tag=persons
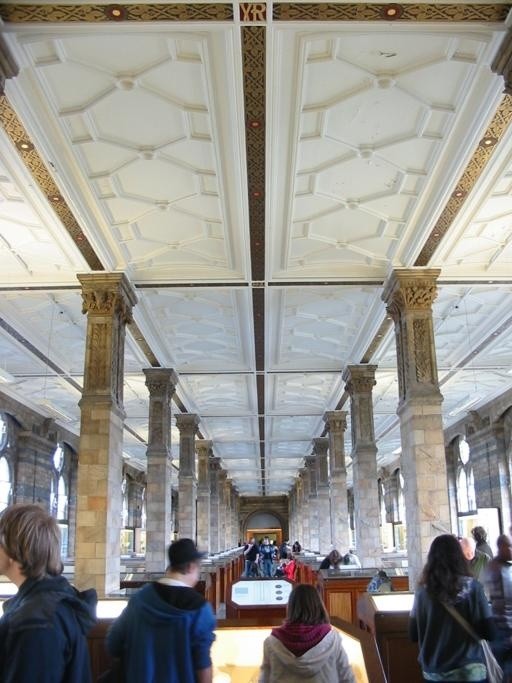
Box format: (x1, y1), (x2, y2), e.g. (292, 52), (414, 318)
(470, 525), (494, 560)
(237, 535), (360, 578)
(408, 534), (498, 682)
(102, 536), (217, 682)
(1, 505), (102, 682)
(367, 569), (395, 592)
(460, 537), (491, 581)
(477, 534), (512, 682)
(257, 583), (359, 682)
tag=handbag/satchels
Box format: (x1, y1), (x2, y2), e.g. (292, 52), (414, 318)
(479, 639), (505, 683)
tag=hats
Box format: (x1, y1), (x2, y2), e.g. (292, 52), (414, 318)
(169, 539), (207, 566)
